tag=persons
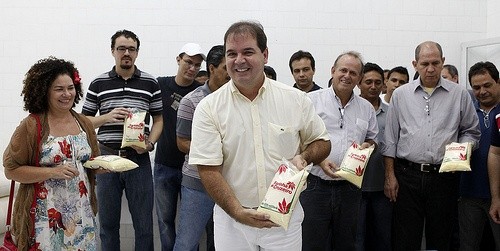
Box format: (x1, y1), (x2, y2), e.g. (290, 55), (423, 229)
(194, 71), (216, 251)
(172, 45), (231, 251)
(264, 66), (276, 81)
(153, 42), (208, 251)
(188, 21), (332, 251)
(289, 41), (500, 251)
(81, 29), (164, 251)
(3, 58), (112, 251)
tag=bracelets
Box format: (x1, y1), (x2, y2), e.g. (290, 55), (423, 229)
(148, 140), (155, 151)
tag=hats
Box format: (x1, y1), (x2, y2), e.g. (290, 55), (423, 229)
(178, 43), (207, 61)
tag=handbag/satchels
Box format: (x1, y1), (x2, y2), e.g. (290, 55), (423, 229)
(0, 230), (40, 251)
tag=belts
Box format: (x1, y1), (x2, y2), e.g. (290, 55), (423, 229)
(99, 143), (137, 157)
(402, 159), (441, 175)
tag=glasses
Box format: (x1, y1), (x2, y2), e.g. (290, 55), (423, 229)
(112, 46), (139, 53)
(179, 55), (202, 69)
